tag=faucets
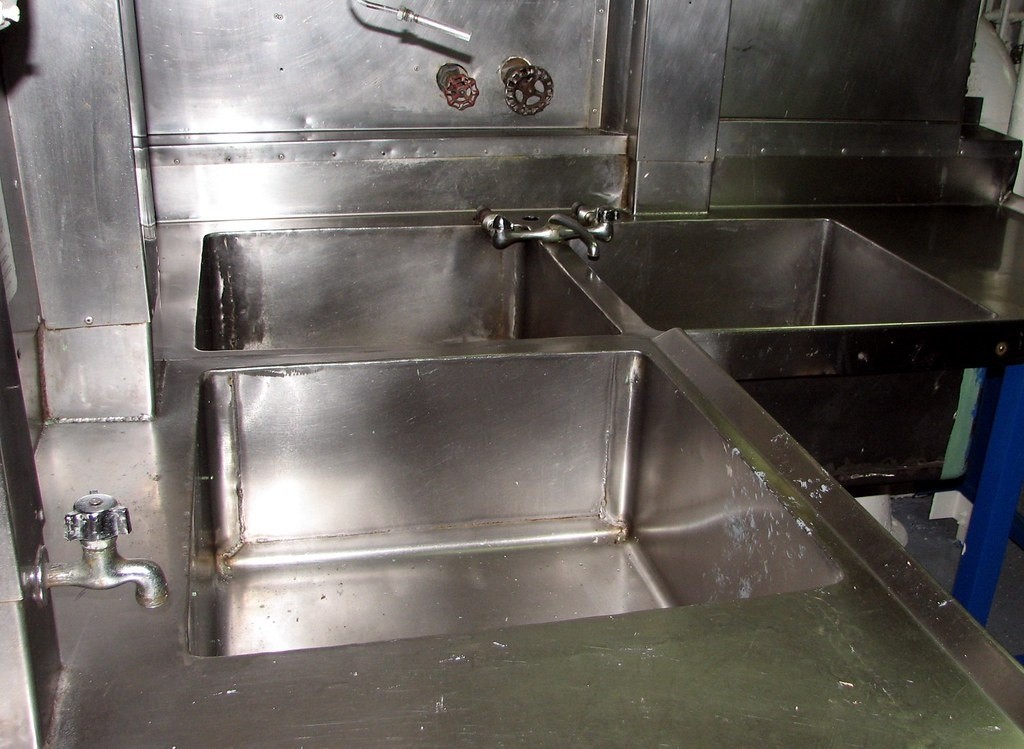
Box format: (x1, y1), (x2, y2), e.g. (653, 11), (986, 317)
(23, 493), (170, 609)
(542, 214), (605, 262)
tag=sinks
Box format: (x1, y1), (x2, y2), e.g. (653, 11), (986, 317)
(159, 338), (878, 669)
(179, 222), (650, 361)
(518, 211), (1013, 497)
